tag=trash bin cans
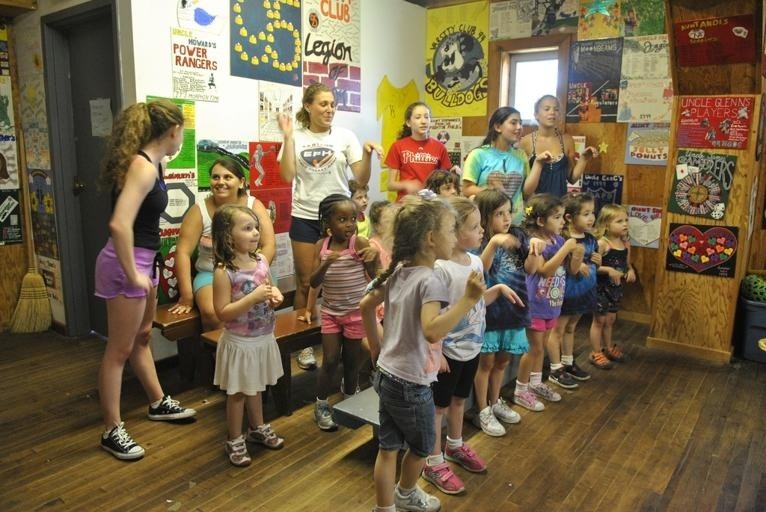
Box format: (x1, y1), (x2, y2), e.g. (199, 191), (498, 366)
(738, 298), (765, 364)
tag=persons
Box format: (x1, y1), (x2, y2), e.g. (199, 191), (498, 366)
(165, 158), (279, 334)
(513, 195), (585, 412)
(91, 94), (198, 464)
(516, 93), (600, 200)
(472, 189), (546, 437)
(461, 106), (557, 234)
(358, 192), (488, 512)
(429, 170), (459, 196)
(347, 180), (392, 325)
(546, 193), (602, 388)
(381, 100), (457, 210)
(588, 202), (636, 368)
(273, 82), (386, 375)
(310, 194), (382, 429)
(209, 203), (286, 470)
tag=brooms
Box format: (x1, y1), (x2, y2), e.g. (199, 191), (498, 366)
(6, 128), (54, 335)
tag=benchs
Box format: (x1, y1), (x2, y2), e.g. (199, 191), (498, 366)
(330, 353), (535, 463)
(199, 302), (321, 418)
(151, 272), (295, 382)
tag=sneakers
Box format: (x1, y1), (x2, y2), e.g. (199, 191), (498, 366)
(421, 460), (465, 495)
(548, 345), (629, 389)
(99, 421), (145, 460)
(225, 434), (252, 466)
(471, 397), (520, 437)
(246, 424), (284, 448)
(147, 395), (197, 421)
(444, 442), (487, 472)
(393, 483), (441, 512)
(314, 398), (338, 430)
(513, 382), (561, 411)
(296, 348), (317, 369)
